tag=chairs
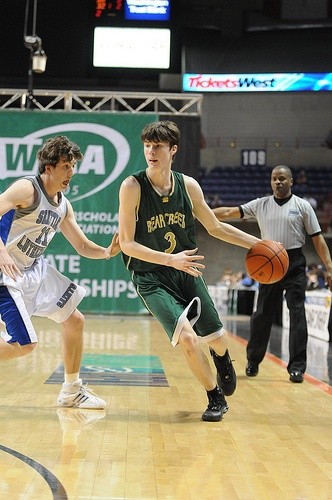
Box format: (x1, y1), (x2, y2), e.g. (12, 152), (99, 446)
(201, 165), (332, 206)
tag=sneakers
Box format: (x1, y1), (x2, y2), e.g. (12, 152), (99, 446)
(210, 349), (236, 397)
(201, 391), (228, 422)
(56, 383), (106, 409)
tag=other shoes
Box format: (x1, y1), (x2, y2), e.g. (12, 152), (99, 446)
(289, 369), (304, 384)
(246, 361), (259, 376)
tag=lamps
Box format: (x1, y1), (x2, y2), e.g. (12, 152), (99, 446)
(31, 46), (48, 73)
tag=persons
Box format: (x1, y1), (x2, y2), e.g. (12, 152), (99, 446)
(305, 264), (329, 291)
(119, 121), (283, 422)
(0, 136), (121, 408)
(302, 194), (317, 210)
(221, 268), (259, 288)
(193, 164), (332, 383)
(295, 168), (307, 185)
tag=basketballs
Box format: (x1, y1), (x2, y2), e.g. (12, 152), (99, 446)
(245, 239), (289, 284)
